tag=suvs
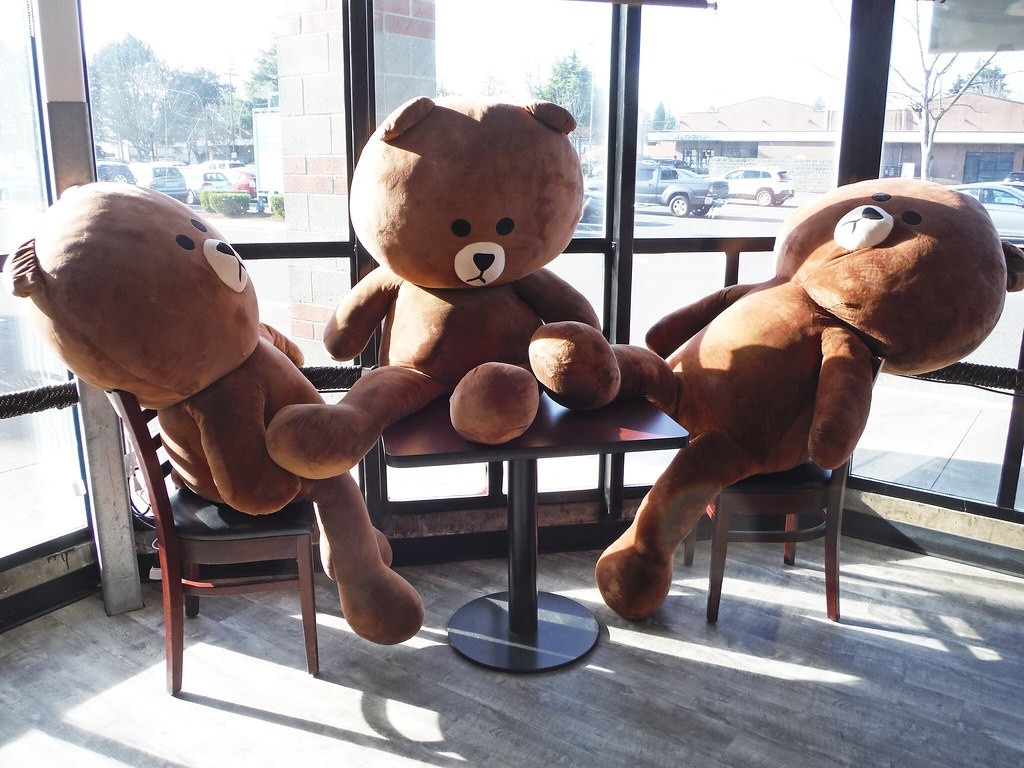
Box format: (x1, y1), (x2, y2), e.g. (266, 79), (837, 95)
(197, 159), (244, 170)
(135, 165), (188, 202)
(703, 168), (796, 207)
(96, 161), (134, 186)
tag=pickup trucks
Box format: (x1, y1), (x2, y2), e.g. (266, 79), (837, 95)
(584, 162), (731, 218)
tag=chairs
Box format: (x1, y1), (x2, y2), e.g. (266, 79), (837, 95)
(685, 355), (885, 621)
(103, 388), (319, 697)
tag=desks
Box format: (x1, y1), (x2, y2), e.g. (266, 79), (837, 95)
(378, 386), (689, 675)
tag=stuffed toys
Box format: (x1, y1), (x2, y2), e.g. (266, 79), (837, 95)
(264, 96), (601, 479)
(527, 177), (1024, 619)
(4, 180), (425, 646)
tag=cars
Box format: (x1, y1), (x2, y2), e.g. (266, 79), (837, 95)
(583, 176), (604, 224)
(946, 182), (1024, 249)
(229, 172), (257, 198)
(184, 171), (232, 204)
(1003, 172), (1024, 190)
